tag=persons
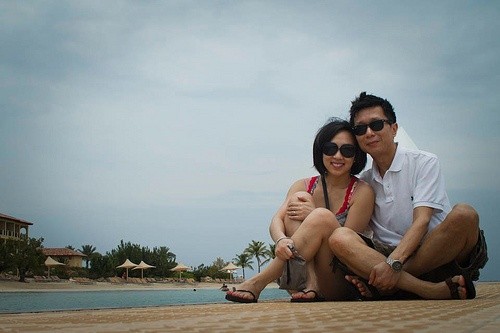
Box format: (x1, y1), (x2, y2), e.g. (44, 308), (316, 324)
(219, 283), (228, 289)
(328, 92), (488, 299)
(226, 118), (378, 302)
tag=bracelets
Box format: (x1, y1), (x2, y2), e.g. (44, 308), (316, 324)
(276, 236), (289, 245)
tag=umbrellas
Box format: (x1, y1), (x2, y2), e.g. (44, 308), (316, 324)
(116, 258), (138, 282)
(130, 260), (157, 279)
(42, 255), (68, 277)
(170, 262), (193, 280)
(219, 262), (246, 279)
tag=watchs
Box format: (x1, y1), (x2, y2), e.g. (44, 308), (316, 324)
(386, 256), (403, 272)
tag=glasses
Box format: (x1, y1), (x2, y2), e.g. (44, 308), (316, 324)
(322, 142), (355, 158)
(351, 120), (388, 136)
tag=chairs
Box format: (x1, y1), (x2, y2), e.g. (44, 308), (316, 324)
(216, 279), (243, 283)
(108, 278), (192, 284)
(34, 276), (66, 282)
(73, 278), (96, 286)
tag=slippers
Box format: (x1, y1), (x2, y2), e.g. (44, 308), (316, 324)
(291, 289), (325, 302)
(352, 273), (392, 301)
(225, 287), (258, 303)
(445, 271), (476, 301)
